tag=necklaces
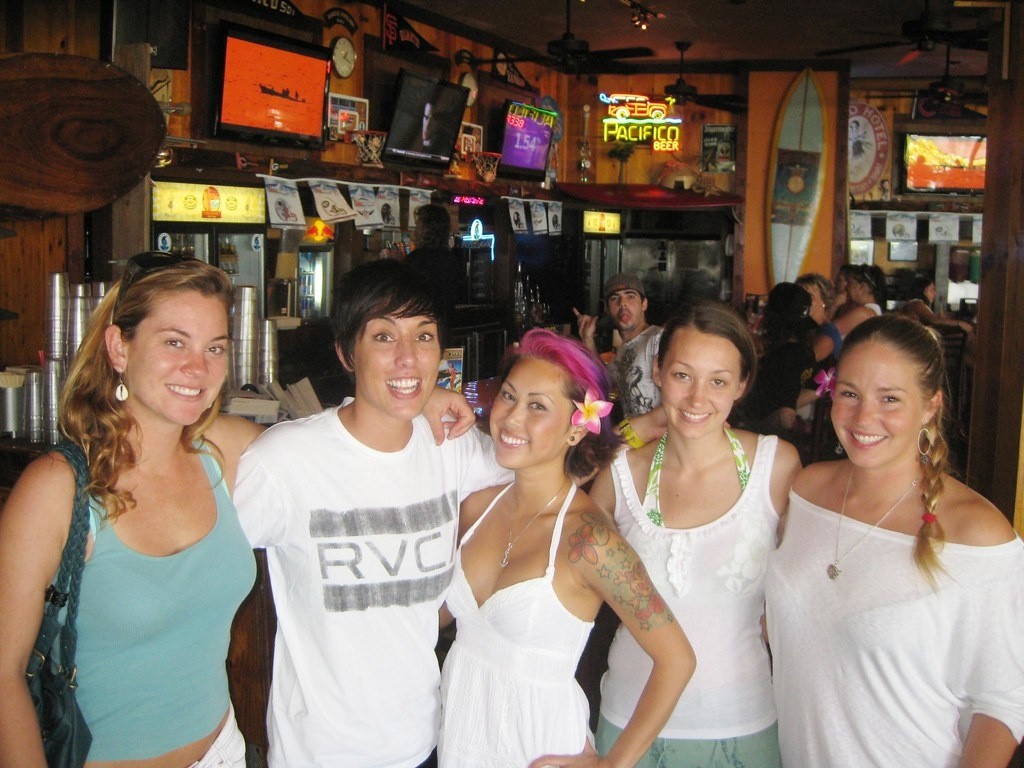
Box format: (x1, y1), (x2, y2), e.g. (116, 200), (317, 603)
(827, 465), (918, 581)
(499, 486), (563, 567)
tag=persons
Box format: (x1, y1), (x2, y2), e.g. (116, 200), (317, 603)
(236, 258), (667, 768)
(0, 250), (476, 768)
(746, 263), (887, 425)
(403, 204), (466, 298)
(573, 273), (665, 417)
(900, 277), (974, 333)
(437, 329), (697, 768)
(589, 300), (802, 768)
(764, 313), (1024, 768)
(420, 97), (451, 156)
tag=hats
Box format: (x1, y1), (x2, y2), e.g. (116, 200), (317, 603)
(768, 281), (812, 307)
(603, 271), (646, 303)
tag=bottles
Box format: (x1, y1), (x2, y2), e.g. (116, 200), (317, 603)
(298, 251), (315, 318)
(218, 233), (239, 274)
(515, 260), (552, 316)
(169, 233), (196, 260)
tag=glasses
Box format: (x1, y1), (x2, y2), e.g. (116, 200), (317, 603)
(111, 250), (206, 321)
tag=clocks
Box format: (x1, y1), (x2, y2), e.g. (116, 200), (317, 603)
(330, 37), (357, 78)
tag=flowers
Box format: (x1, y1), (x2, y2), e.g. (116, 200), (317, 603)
(571, 388), (614, 434)
(814, 368), (835, 397)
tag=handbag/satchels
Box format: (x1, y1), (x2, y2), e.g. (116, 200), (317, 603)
(27, 442), (93, 768)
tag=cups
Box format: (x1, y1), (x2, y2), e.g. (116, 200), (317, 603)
(27, 270), (111, 445)
(0, 387), (24, 432)
(221, 286), (279, 392)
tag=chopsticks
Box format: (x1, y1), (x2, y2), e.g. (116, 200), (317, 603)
(0, 371), (25, 387)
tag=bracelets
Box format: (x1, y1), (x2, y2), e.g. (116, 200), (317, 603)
(957, 320), (960, 326)
(618, 416), (645, 449)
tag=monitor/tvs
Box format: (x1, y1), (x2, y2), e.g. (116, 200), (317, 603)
(381, 66), (470, 170)
(496, 99), (554, 182)
(900, 133), (987, 196)
(213, 19), (332, 153)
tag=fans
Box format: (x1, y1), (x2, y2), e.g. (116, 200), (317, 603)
(865, 43), (990, 119)
(814, 1), (989, 55)
(607, 41), (748, 114)
(476, 0), (654, 73)
